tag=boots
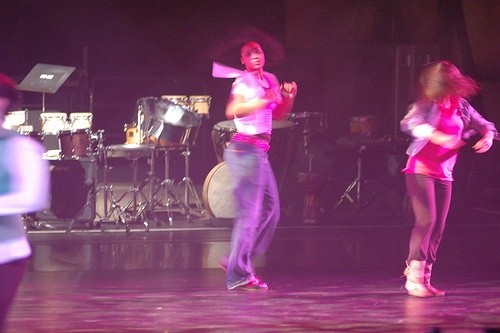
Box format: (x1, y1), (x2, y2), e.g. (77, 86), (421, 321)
(403, 260), (433, 297)
(424, 262), (445, 296)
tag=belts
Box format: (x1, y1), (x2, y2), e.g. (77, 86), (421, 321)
(233, 133), (270, 152)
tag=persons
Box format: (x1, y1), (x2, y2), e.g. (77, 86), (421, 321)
(0, 74), (52, 333)
(400, 60), (499, 297)
(218, 27), (298, 295)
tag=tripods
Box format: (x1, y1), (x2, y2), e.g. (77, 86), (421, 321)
(331, 149), (382, 212)
(87, 135), (205, 234)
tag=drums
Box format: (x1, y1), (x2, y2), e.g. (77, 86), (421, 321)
(202, 159), (241, 220)
(57, 128), (94, 158)
(51, 159), (88, 219)
(212, 119), (294, 197)
(286, 109), (330, 174)
(14, 127), (45, 146)
(191, 96), (209, 118)
(162, 94), (188, 110)
(124, 125), (184, 150)
(134, 98), (201, 147)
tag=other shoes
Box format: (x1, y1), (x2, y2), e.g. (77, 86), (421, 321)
(221, 256), (262, 281)
(234, 279), (269, 291)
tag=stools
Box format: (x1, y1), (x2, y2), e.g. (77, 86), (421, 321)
(106, 144), (155, 234)
